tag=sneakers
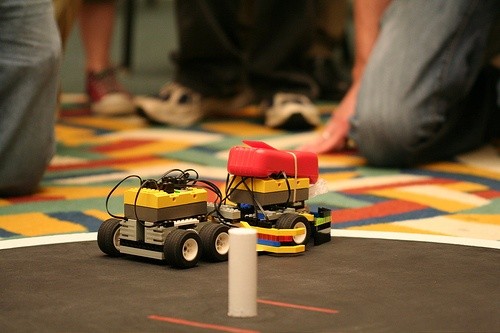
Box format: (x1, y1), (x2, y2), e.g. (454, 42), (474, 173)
(265, 92), (320, 128)
(133, 81), (253, 127)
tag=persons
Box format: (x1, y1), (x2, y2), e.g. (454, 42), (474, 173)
(50, 0), (136, 122)
(0, 0), (63, 197)
(134, 0), (356, 130)
(295, 0), (500, 169)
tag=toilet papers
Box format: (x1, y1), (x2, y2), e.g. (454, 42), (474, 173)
(228, 227), (258, 318)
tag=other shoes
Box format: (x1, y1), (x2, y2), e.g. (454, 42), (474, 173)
(87, 70), (135, 115)
